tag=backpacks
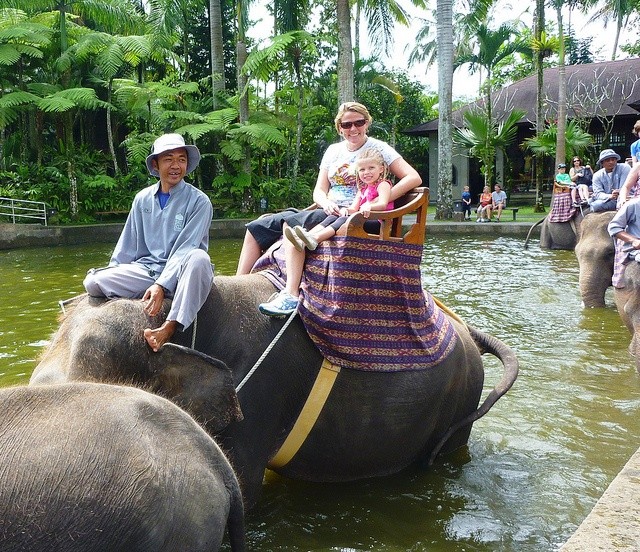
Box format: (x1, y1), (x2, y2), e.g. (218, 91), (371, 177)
(576, 168), (592, 185)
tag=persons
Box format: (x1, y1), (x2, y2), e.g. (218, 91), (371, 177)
(569, 155), (595, 207)
(284, 148), (396, 254)
(461, 185), (473, 222)
(235, 101), (424, 321)
(606, 197), (640, 265)
(84, 131), (214, 354)
(588, 149), (637, 213)
(483, 184), (508, 223)
(475, 186), (493, 223)
(612, 162), (640, 288)
(555, 163), (588, 208)
(629, 119), (640, 167)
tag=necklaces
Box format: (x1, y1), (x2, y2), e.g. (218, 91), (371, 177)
(346, 137), (368, 153)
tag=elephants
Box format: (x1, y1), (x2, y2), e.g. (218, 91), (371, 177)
(524, 205), (589, 250)
(0, 380), (247, 552)
(30, 273), (520, 520)
(575, 211), (619, 308)
(613, 260), (640, 373)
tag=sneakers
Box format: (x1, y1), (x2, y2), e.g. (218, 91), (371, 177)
(258, 289), (299, 316)
(495, 218), (499, 222)
(484, 219), (491, 221)
(284, 227), (305, 251)
(294, 226), (318, 251)
(477, 218), (480, 223)
(480, 218), (486, 222)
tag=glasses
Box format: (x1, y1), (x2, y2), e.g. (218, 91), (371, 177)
(573, 159), (580, 162)
(339, 119), (368, 129)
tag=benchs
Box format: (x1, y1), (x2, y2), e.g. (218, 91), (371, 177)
(503, 207), (519, 220)
(300, 186), (430, 246)
(553, 180), (571, 194)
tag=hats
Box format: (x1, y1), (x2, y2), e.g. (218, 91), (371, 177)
(145, 133), (201, 177)
(558, 163), (566, 168)
(596, 149), (621, 164)
